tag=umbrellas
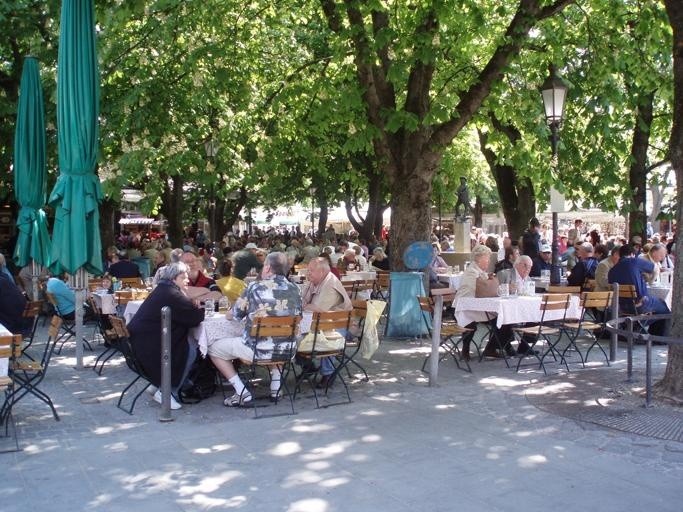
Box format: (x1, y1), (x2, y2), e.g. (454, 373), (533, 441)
(46, 1), (106, 279)
(11, 54), (51, 267)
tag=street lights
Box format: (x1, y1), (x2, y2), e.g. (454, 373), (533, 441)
(204, 136), (221, 243)
(537, 59), (567, 286)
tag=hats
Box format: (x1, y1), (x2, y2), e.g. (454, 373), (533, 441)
(539, 244), (552, 253)
(243, 243), (259, 251)
(576, 242), (593, 253)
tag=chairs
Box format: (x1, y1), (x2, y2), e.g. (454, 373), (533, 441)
(560, 291), (613, 368)
(431, 287), (481, 360)
(583, 279), (596, 289)
(608, 284), (653, 346)
(511, 294), (571, 374)
(548, 285), (581, 293)
(416, 296), (472, 373)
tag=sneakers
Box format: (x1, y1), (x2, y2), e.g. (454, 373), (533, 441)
(506, 352), (525, 359)
(145, 384), (158, 396)
(152, 389), (182, 410)
(303, 365), (320, 373)
(317, 375), (334, 388)
(460, 354), (471, 362)
(516, 349), (541, 357)
(482, 351), (505, 360)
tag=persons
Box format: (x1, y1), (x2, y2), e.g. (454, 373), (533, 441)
(454, 176), (470, 222)
(429, 216), (680, 314)
(451, 244), (510, 362)
(18, 260), (57, 317)
(206, 251), (303, 407)
(293, 256), (357, 388)
(0, 254), (35, 338)
(495, 254), (542, 357)
(120, 261), (205, 411)
(46, 270), (113, 348)
(609, 244), (670, 345)
(103, 223), (391, 312)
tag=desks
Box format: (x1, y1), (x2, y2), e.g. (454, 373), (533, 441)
(194, 310), (313, 403)
(438, 271), (465, 288)
(646, 282), (672, 313)
(453, 296), (583, 368)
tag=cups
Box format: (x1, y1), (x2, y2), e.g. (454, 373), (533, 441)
(251, 267), (256, 277)
(517, 281), (535, 296)
(660, 271), (668, 286)
(541, 270), (550, 280)
(204, 298), (214, 318)
(219, 296), (228, 314)
(455, 264), (459, 273)
(497, 283), (509, 299)
(447, 266), (452, 275)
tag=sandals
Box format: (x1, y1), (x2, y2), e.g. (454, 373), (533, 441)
(269, 387), (283, 401)
(223, 391), (255, 408)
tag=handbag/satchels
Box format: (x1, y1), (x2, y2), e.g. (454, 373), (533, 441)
(476, 276), (501, 298)
(360, 300), (387, 361)
(177, 358), (216, 405)
